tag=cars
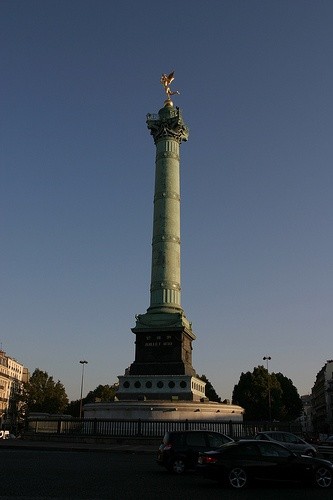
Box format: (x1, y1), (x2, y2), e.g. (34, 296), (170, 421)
(254, 431), (318, 457)
(197, 440), (333, 493)
(157, 430), (238, 477)
(317, 436), (333, 458)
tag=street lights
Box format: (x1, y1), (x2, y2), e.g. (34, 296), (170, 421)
(78, 360), (89, 419)
(263, 356), (272, 420)
